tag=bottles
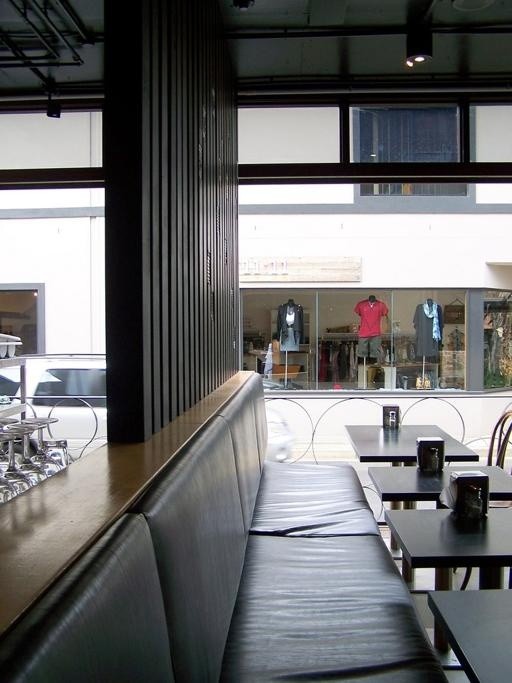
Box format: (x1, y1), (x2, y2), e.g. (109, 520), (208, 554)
(427, 448), (439, 473)
(387, 411), (396, 431)
(465, 484), (484, 521)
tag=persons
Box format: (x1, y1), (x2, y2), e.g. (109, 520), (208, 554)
(413, 298), (443, 357)
(277, 298), (304, 352)
(354, 294), (388, 357)
(263, 333), (280, 379)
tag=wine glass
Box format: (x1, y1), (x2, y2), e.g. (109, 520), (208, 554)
(0, 418), (62, 504)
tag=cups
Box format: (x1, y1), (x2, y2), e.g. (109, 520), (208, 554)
(44, 440), (69, 468)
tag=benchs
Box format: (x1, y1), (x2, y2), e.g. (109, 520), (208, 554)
(212, 372), (380, 536)
(0, 514), (177, 683)
(141, 414), (438, 683)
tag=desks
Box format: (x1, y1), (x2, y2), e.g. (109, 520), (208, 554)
(345, 425), (512, 683)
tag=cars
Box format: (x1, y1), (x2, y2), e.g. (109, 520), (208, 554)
(0, 348), (108, 463)
(261, 376), (303, 392)
